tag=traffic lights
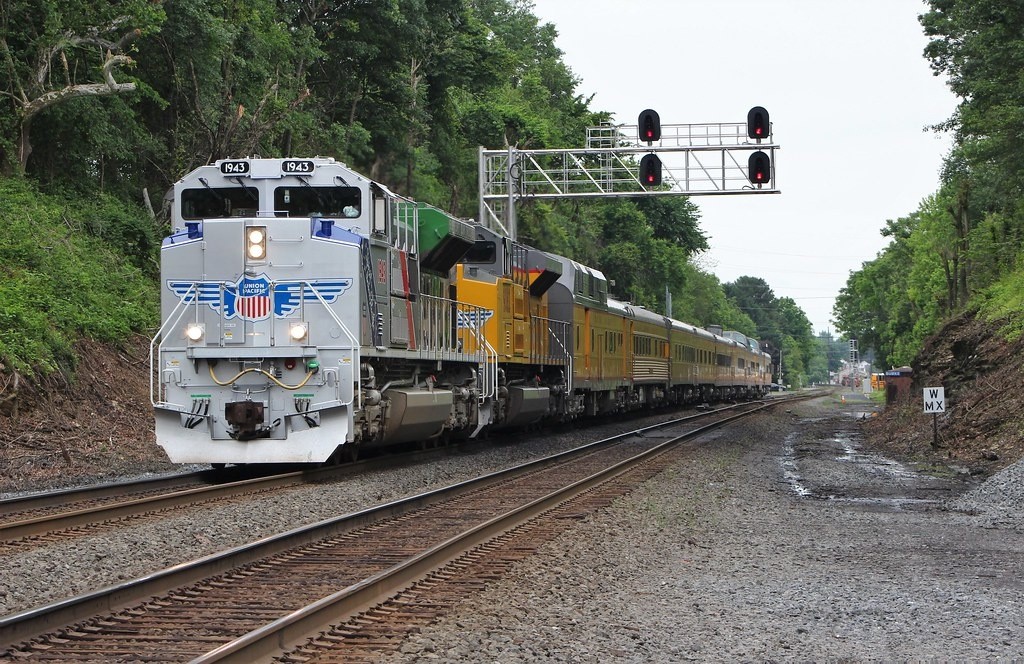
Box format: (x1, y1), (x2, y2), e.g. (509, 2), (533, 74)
(638, 109), (660, 142)
(639, 154), (662, 187)
(748, 152), (770, 183)
(748, 106), (769, 138)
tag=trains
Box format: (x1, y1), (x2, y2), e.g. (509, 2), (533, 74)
(150, 154), (772, 470)
(872, 373), (886, 388)
